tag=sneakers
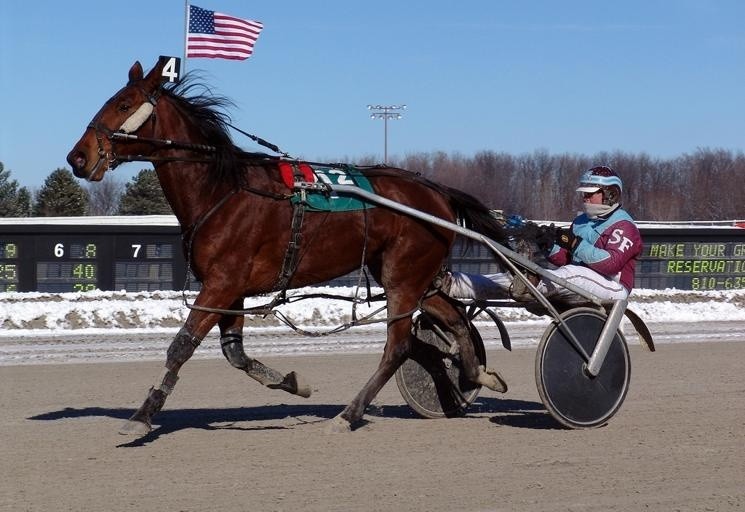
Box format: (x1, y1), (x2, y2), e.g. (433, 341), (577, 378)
(512, 240), (539, 293)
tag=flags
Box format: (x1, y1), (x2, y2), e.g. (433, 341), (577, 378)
(185, 5), (264, 61)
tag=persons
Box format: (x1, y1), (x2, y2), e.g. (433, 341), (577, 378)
(431, 164), (645, 306)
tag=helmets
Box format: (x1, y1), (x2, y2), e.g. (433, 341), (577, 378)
(576, 166), (623, 206)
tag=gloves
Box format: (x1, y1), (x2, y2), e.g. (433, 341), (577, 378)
(556, 227), (582, 252)
(536, 224), (555, 256)
